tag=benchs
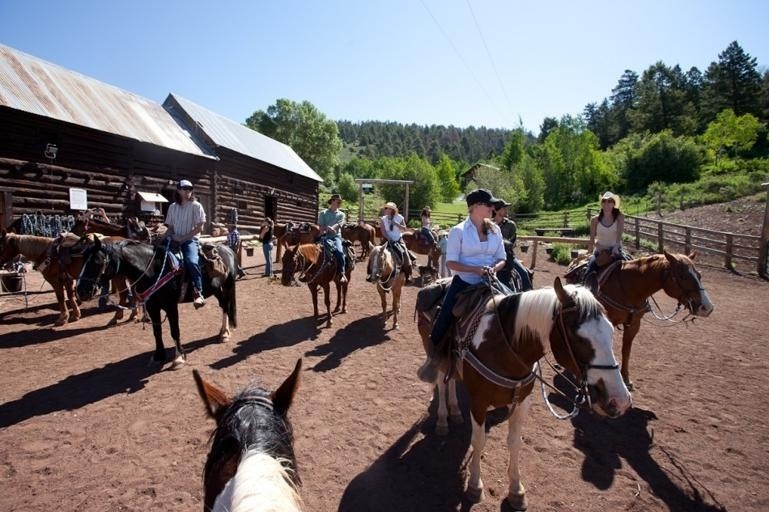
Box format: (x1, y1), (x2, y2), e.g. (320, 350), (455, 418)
(535, 228), (572, 245)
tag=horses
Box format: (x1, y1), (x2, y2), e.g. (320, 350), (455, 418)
(75, 233), (237, 370)
(280, 241), (353, 328)
(366, 240), (405, 329)
(566, 249), (714, 391)
(417, 276), (633, 510)
(70, 216), (149, 322)
(0, 227), (139, 328)
(192, 357), (304, 511)
(273, 221), (321, 250)
(402, 230), (441, 279)
(341, 222), (376, 260)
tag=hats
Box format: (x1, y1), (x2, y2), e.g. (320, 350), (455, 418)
(383, 202), (399, 214)
(423, 206), (432, 212)
(328, 195), (342, 204)
(177, 179), (194, 189)
(466, 189), (511, 208)
(599, 191), (620, 208)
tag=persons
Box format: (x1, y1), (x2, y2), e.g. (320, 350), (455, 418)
(367, 202), (412, 283)
(161, 179), (204, 306)
(222, 224), (245, 279)
(116, 212), (151, 244)
(317, 195), (348, 282)
(581, 192), (630, 285)
(421, 206), (434, 245)
(428, 190), (514, 358)
(433, 230), (452, 278)
(259, 217), (274, 276)
(491, 200), (517, 290)
